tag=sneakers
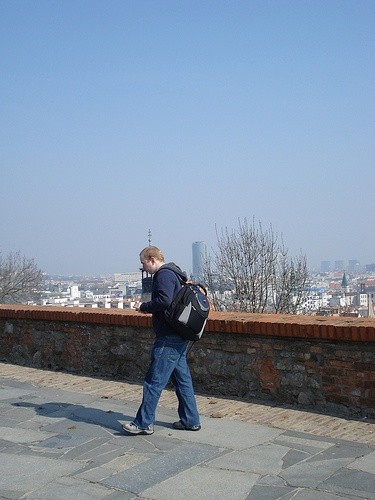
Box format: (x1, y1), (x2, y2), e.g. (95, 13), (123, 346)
(122, 423), (154, 434)
(173, 420), (201, 431)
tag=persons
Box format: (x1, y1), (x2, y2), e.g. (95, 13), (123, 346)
(122, 246), (201, 434)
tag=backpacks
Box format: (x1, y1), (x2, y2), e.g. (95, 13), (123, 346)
(154, 272), (210, 341)
